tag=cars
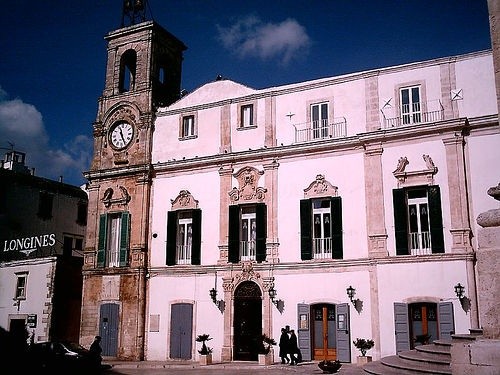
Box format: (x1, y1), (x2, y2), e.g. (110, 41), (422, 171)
(35, 342), (83, 362)
(63, 343), (88, 357)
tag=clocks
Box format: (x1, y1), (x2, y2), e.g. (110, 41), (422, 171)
(108, 120), (134, 151)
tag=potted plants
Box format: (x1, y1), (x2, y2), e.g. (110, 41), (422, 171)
(353, 338), (374, 366)
(194, 333), (214, 366)
(257, 333), (277, 366)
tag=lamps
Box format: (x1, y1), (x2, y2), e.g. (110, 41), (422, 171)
(346, 286), (359, 303)
(269, 286), (278, 303)
(454, 282), (465, 299)
(209, 287), (221, 306)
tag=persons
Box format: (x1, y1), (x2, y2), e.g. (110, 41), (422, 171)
(89, 336), (103, 365)
(279, 328), (290, 364)
(289, 330), (299, 365)
